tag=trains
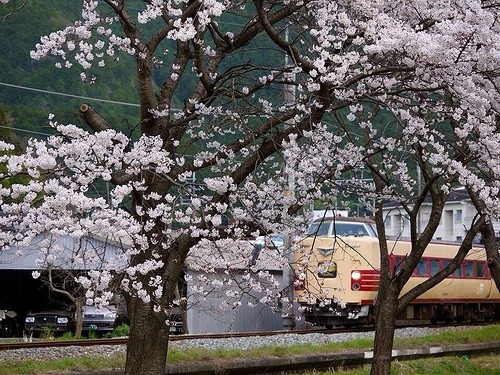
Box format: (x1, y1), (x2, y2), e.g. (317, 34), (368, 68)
(294, 237), (500, 329)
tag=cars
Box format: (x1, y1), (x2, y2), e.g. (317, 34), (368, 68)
(81, 301), (125, 330)
(26, 301), (74, 333)
(165, 295), (185, 332)
